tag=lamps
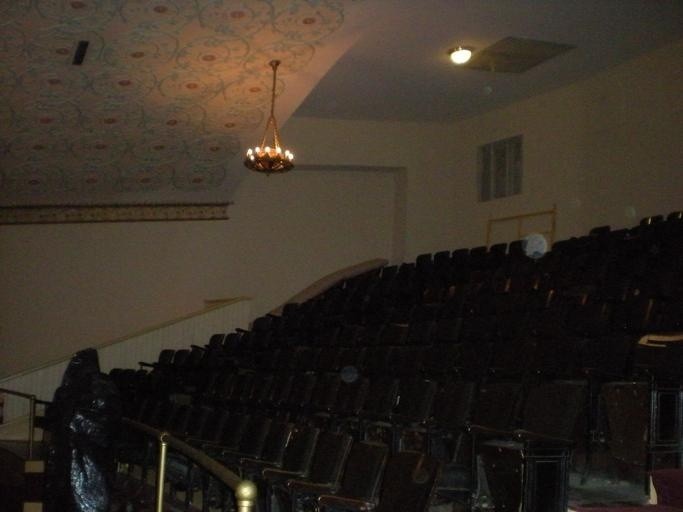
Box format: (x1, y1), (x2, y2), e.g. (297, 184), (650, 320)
(243, 59), (295, 173)
(448, 45), (473, 65)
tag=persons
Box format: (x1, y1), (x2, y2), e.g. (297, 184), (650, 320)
(37, 346), (119, 512)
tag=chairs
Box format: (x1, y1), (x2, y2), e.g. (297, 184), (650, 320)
(100, 212), (683, 512)
